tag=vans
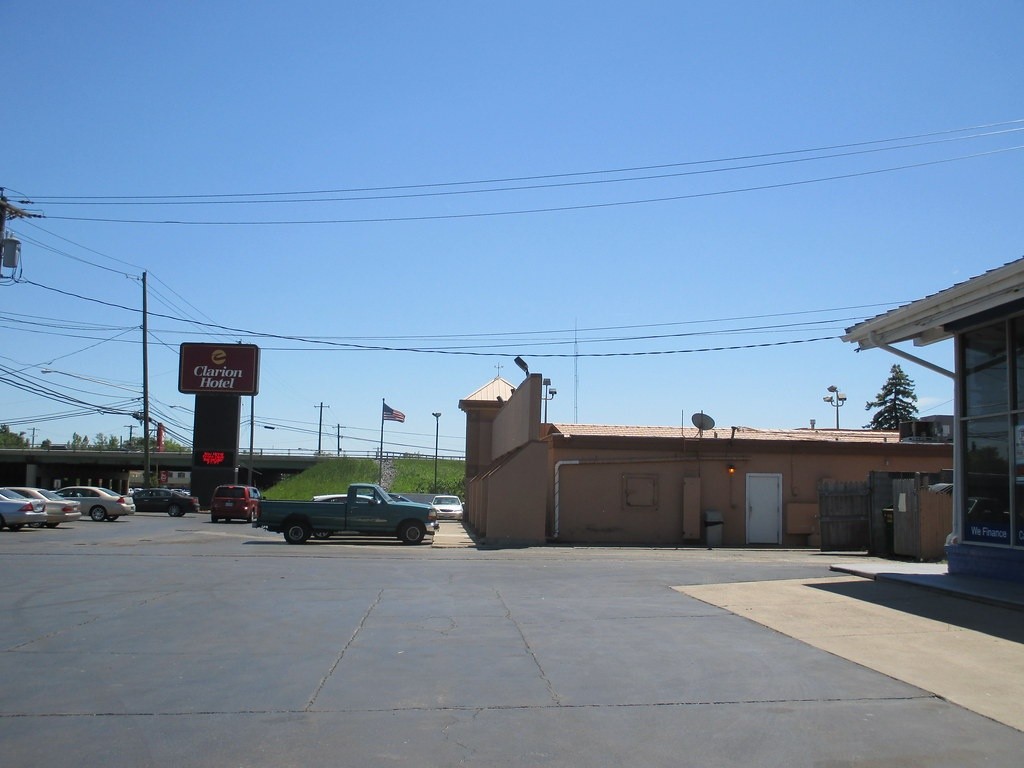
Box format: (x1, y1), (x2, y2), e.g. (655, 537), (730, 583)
(209, 485), (259, 523)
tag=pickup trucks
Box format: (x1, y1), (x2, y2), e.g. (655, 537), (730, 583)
(252, 482), (438, 545)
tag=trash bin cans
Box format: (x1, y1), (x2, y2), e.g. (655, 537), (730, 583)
(882, 504), (894, 559)
(704, 510), (723, 548)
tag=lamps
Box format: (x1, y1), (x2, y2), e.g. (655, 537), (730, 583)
(728, 464), (736, 475)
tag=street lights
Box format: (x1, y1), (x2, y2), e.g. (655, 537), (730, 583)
(823, 386), (847, 429)
(432, 412), (441, 493)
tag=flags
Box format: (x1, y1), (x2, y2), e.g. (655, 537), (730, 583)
(383, 403), (405, 423)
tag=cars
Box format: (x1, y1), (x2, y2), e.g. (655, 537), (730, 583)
(2, 486), (82, 528)
(429, 494), (465, 521)
(0, 487), (48, 530)
(132, 488), (200, 517)
(56, 485), (137, 522)
(310, 493), (382, 504)
(364, 492), (413, 502)
